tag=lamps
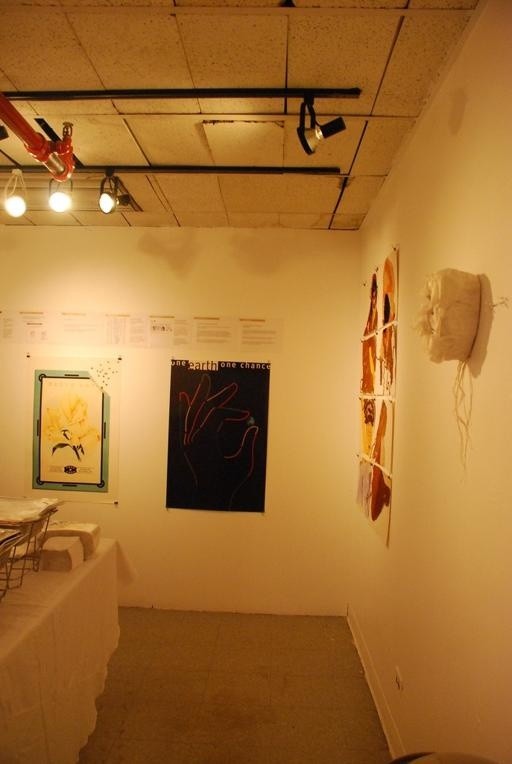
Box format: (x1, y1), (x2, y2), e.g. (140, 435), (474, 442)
(293, 96), (348, 157)
(98, 168), (132, 216)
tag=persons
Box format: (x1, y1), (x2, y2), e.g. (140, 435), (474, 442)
(179, 373), (259, 510)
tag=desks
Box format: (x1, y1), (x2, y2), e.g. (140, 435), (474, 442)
(0, 536), (120, 764)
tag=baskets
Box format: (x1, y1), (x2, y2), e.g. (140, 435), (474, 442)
(1, 508), (58, 598)
